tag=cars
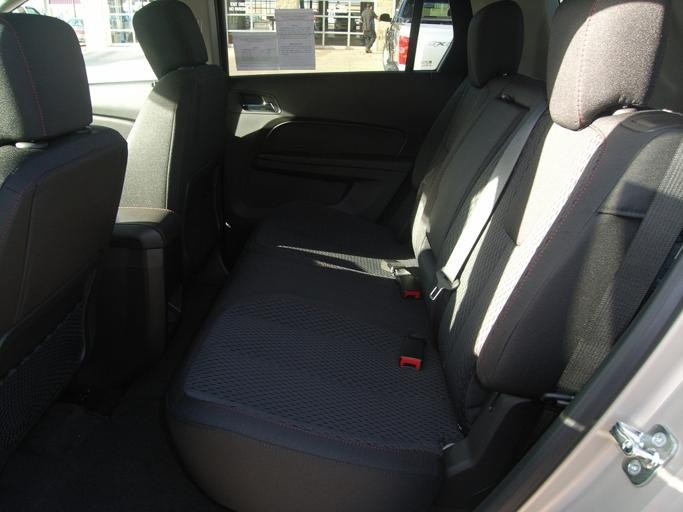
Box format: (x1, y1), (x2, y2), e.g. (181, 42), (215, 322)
(332, 13), (356, 33)
(65, 18), (87, 49)
(379, 3), (456, 72)
(311, 11), (322, 32)
(12, 6), (46, 17)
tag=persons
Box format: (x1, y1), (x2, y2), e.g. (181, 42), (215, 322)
(359, 2), (380, 53)
